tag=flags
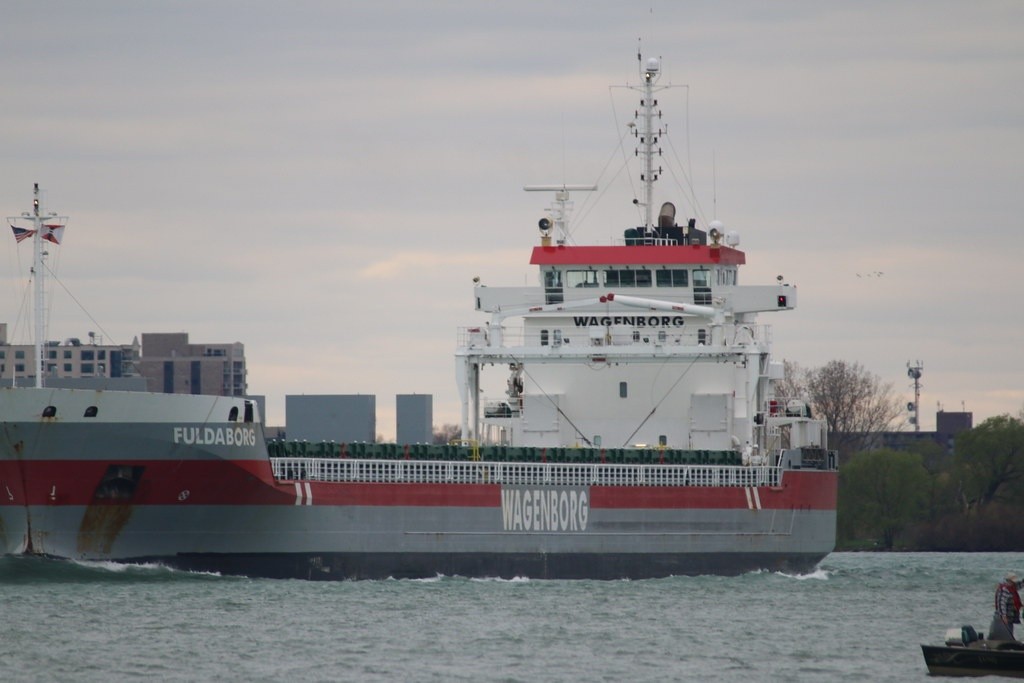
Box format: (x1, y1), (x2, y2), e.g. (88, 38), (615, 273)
(40, 224), (67, 244)
(11, 224), (35, 244)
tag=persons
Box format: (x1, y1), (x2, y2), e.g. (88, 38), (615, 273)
(994, 571), (1024, 639)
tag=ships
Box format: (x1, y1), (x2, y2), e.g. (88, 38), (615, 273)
(0, 36), (841, 584)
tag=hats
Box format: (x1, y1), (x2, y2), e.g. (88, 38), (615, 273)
(1005, 572), (1019, 583)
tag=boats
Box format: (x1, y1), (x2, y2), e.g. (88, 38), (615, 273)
(919, 625), (1024, 679)
